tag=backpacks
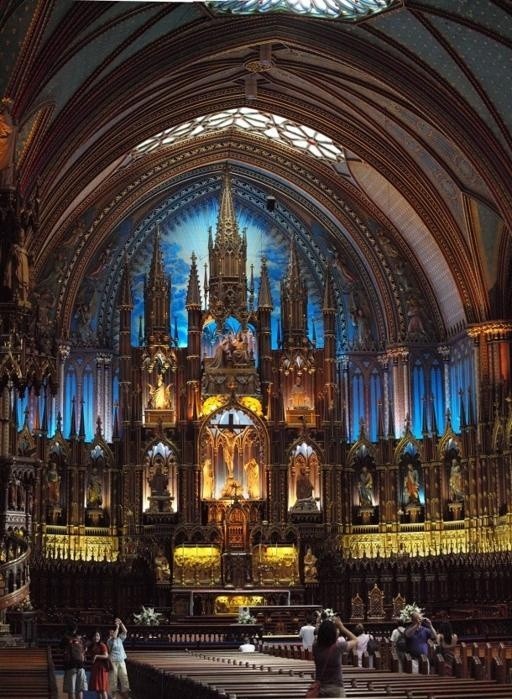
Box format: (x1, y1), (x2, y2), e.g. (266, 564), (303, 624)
(396, 628), (408, 652)
(63, 635), (84, 666)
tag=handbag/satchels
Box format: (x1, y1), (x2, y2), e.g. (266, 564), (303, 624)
(103, 658), (112, 671)
(367, 634), (380, 656)
(305, 680), (320, 698)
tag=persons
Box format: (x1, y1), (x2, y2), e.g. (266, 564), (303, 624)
(107, 618), (130, 699)
(240, 607), (249, 617)
(298, 616), (458, 675)
(245, 459), (260, 499)
(358, 467), (373, 506)
(202, 458), (214, 499)
(313, 611), (359, 697)
(147, 374), (172, 404)
(60, 620), (88, 698)
(448, 459), (462, 501)
(88, 468), (102, 505)
(88, 630), (109, 699)
(46, 463), (61, 504)
(296, 467), (314, 499)
(404, 463), (420, 499)
